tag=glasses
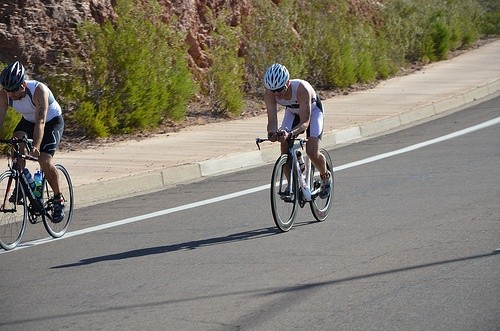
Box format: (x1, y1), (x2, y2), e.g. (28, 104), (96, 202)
(3, 83), (22, 93)
(270, 84), (286, 93)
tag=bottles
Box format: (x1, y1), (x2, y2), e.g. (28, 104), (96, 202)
(34, 171), (43, 199)
(23, 168), (35, 189)
(296, 150), (305, 170)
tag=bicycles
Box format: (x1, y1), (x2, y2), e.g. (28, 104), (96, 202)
(0, 134), (74, 250)
(255, 127), (335, 232)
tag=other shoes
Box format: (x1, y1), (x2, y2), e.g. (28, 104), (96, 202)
(319, 171), (333, 199)
(52, 194), (65, 223)
(9, 187), (23, 202)
(280, 186), (294, 200)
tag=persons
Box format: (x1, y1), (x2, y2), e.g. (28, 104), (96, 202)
(0, 61), (66, 223)
(264, 63), (332, 198)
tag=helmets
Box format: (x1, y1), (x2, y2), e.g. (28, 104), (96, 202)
(0, 61), (26, 90)
(264, 63), (289, 90)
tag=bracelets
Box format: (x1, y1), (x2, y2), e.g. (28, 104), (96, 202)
(289, 131), (293, 139)
